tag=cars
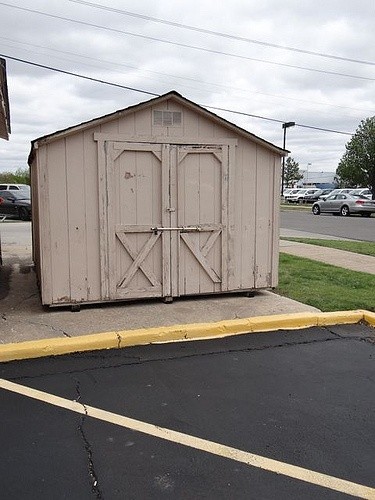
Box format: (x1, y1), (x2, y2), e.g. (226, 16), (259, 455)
(282, 188), (372, 204)
(0, 190), (31, 221)
(311, 193), (375, 217)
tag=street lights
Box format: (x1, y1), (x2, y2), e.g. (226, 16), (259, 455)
(281, 121), (295, 196)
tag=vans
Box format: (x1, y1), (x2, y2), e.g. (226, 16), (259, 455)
(0, 184), (30, 191)
